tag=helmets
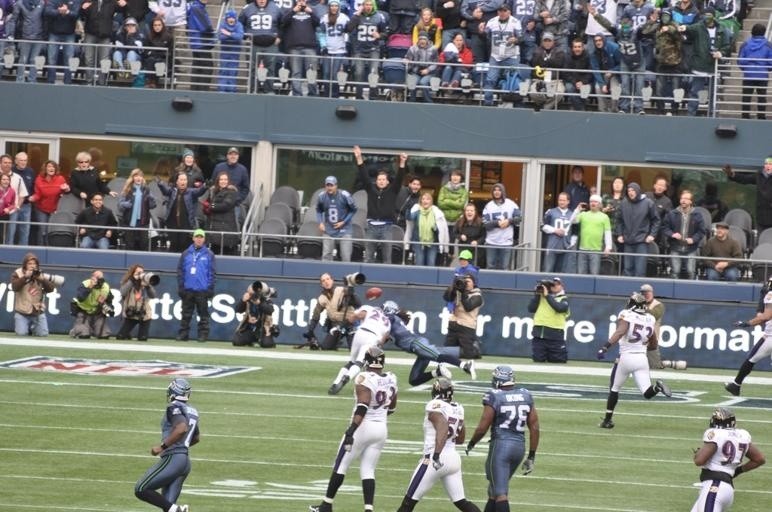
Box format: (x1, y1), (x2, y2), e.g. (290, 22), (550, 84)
(628, 292), (646, 308)
(494, 365), (517, 386)
(710, 408), (734, 427)
(167, 378), (189, 401)
(381, 301), (399, 317)
(366, 287), (382, 301)
(365, 348), (385, 369)
(432, 378), (455, 402)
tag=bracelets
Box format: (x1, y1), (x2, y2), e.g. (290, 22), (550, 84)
(734, 465), (744, 477)
(468, 440), (475, 448)
(528, 450), (535, 460)
(160, 441), (168, 450)
(433, 452), (440, 460)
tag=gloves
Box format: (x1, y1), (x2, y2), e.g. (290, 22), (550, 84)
(345, 436), (353, 448)
(432, 457), (442, 470)
(522, 458), (535, 476)
(596, 348), (608, 361)
(467, 444), (474, 453)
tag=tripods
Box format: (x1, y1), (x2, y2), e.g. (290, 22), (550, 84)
(334, 292), (359, 352)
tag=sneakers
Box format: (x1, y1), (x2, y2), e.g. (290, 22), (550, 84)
(329, 375), (350, 394)
(199, 331), (209, 341)
(598, 418), (613, 427)
(673, 362), (686, 372)
(655, 379), (671, 397)
(432, 365), (451, 379)
(308, 505), (331, 510)
(724, 382), (741, 395)
(462, 361), (476, 381)
(177, 332), (187, 340)
(177, 504), (188, 511)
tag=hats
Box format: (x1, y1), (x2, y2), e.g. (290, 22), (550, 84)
(184, 147), (194, 154)
(326, 176), (337, 185)
(640, 284), (654, 292)
(194, 228), (205, 236)
(543, 32), (552, 38)
(716, 221), (727, 225)
(457, 250), (471, 259)
(228, 147), (237, 152)
(418, 30), (428, 37)
(497, 3), (510, 7)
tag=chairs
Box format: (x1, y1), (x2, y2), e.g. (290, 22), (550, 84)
(260, 187), (405, 263)
(46, 177), (170, 251)
(694, 207), (772, 283)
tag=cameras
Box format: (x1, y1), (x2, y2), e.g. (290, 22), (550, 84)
(344, 272), (366, 288)
(95, 278), (105, 289)
(138, 272), (161, 288)
(453, 279), (468, 293)
(253, 281), (277, 298)
(32, 269), (41, 277)
(300, 4), (307, 11)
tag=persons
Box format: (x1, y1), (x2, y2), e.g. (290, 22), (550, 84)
(689, 407), (766, 512)
(541, 158), (771, 280)
(308, 272), (477, 394)
(69, 270), (115, 340)
(464, 366), (541, 512)
(528, 277), (571, 364)
(11, 252), (54, 335)
(726, 282), (772, 397)
(309, 345), (398, 512)
(117, 264), (156, 341)
(232, 284), (275, 347)
(598, 284), (672, 428)
(134, 378), (200, 512)
(444, 250), (483, 358)
(177, 229), (217, 342)
(0, 145), (251, 257)
(397, 376), (483, 512)
(737, 24), (772, 119)
(317, 144), (522, 268)
(0, 0), (751, 116)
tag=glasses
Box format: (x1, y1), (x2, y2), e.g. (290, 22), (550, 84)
(76, 159), (90, 163)
(124, 23), (136, 25)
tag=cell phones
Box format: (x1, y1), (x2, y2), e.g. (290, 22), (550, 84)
(581, 204), (588, 209)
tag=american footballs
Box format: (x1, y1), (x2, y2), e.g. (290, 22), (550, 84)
(365, 287), (383, 301)
